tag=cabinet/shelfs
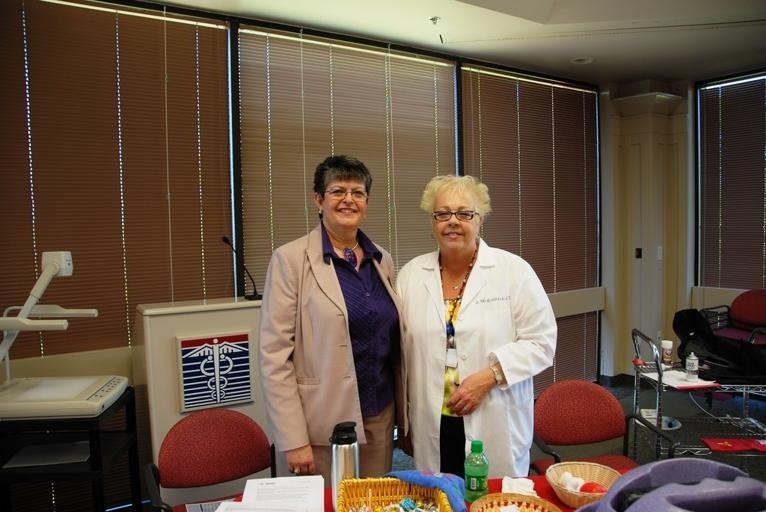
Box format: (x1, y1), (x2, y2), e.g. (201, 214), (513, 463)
(631, 327), (766, 472)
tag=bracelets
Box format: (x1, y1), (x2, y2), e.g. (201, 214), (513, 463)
(490, 366), (502, 385)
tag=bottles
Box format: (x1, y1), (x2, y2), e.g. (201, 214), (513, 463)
(463, 441), (489, 502)
(684, 351), (699, 383)
(327, 420), (360, 512)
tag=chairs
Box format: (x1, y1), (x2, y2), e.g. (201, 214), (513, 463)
(144, 407), (276, 512)
(700, 288), (766, 346)
(530, 380), (644, 479)
(672, 308), (766, 409)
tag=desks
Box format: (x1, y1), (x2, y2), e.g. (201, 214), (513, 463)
(1, 388), (142, 512)
(321, 471), (675, 512)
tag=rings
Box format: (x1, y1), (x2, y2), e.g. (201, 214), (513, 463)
(293, 469), (300, 473)
(463, 407), (469, 412)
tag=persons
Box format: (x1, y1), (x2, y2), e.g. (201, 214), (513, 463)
(258, 153), (410, 489)
(395, 175), (559, 482)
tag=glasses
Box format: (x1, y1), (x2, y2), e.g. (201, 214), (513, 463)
(432, 208), (480, 221)
(322, 187), (369, 199)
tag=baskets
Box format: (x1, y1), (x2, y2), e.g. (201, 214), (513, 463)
(544, 458), (625, 509)
(469, 491), (561, 512)
(338, 473), (451, 512)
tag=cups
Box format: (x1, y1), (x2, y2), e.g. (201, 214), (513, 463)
(660, 339), (673, 364)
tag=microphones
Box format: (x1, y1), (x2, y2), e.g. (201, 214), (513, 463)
(221, 235), (262, 300)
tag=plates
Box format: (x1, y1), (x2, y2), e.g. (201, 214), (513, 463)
(635, 416), (681, 431)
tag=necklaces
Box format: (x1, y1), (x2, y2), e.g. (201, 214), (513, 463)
(446, 281), (461, 291)
(332, 242), (359, 268)
